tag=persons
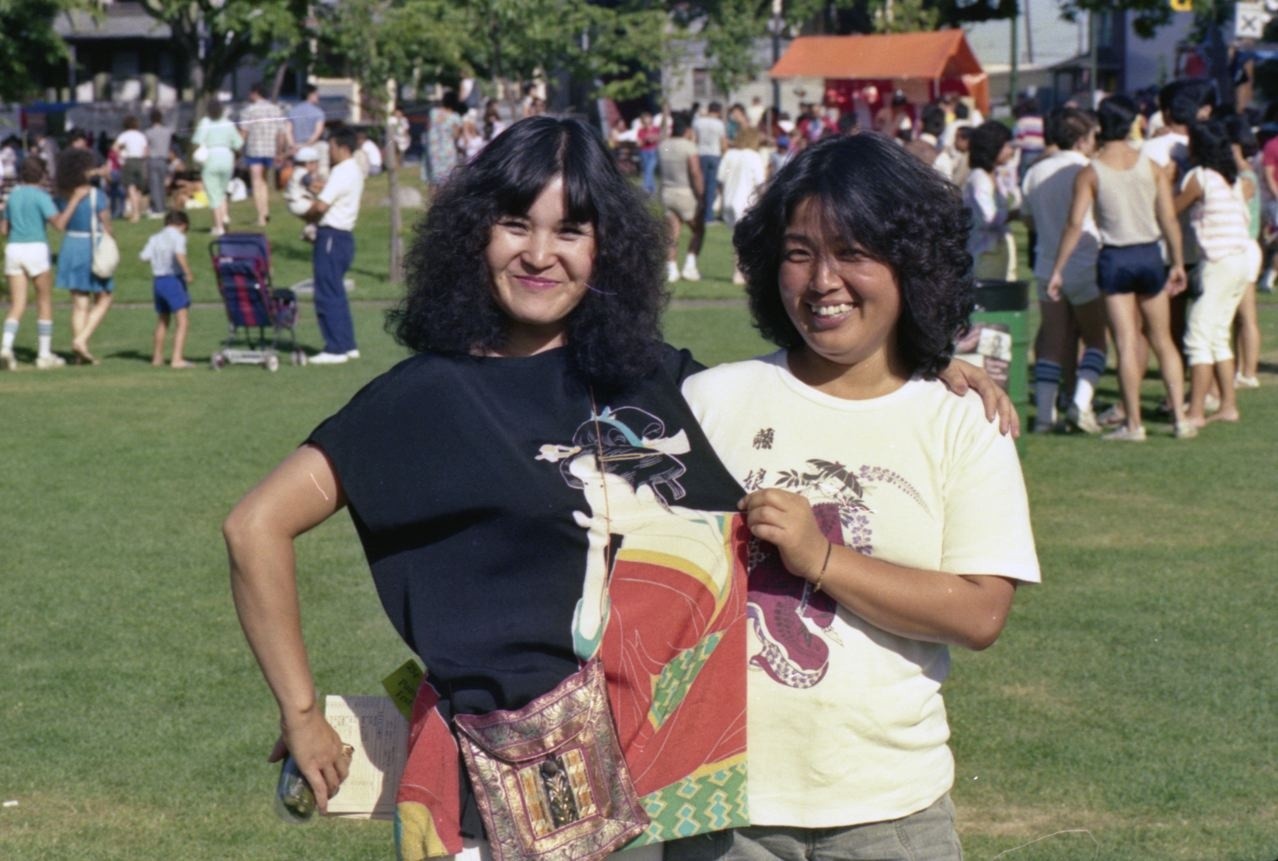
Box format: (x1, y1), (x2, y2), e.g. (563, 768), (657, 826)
(224, 115), (1023, 861)
(0, 71), (1277, 443)
(686, 131), (1046, 861)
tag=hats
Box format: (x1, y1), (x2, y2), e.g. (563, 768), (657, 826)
(293, 147), (320, 163)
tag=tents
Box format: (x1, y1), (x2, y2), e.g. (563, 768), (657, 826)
(770, 30), (986, 144)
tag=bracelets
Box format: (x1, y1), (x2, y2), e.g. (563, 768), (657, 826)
(812, 540), (832, 592)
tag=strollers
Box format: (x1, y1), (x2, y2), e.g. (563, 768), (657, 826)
(208, 232), (311, 373)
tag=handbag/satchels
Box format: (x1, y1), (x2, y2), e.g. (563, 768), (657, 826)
(424, 662), (653, 861)
(91, 234), (120, 277)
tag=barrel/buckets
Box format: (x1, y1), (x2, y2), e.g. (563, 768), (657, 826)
(968, 279), (1033, 457)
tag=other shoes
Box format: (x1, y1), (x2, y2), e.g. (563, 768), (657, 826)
(1102, 425), (1146, 442)
(70, 342), (100, 366)
(35, 353), (66, 369)
(1174, 422), (1197, 441)
(0, 347), (18, 370)
(1183, 393), (1220, 414)
(667, 272), (680, 283)
(731, 272), (746, 285)
(1066, 403), (1102, 434)
(210, 216), (230, 235)
(1096, 403), (1126, 425)
(307, 348), (359, 363)
(1233, 372), (1259, 389)
(682, 267), (701, 281)
